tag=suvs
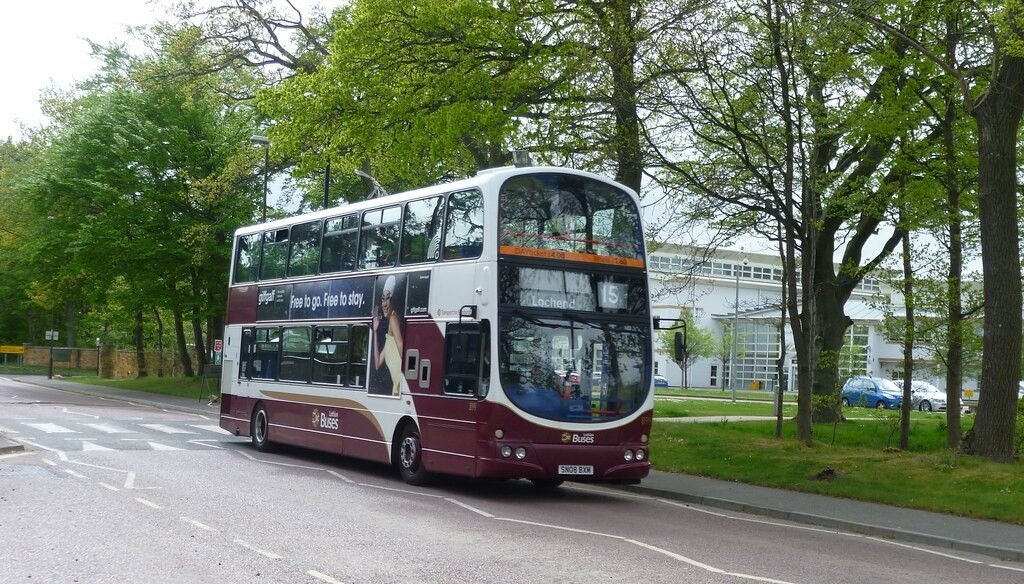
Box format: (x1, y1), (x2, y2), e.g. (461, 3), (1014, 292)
(891, 380), (963, 413)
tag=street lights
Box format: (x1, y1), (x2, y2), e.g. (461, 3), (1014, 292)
(732, 251), (749, 402)
(249, 135), (270, 223)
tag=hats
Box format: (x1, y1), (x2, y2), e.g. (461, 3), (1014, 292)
(383, 276), (396, 298)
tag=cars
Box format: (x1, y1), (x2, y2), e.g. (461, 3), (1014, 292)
(841, 376), (903, 410)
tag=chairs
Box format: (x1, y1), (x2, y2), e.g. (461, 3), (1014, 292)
(249, 261), (306, 280)
(279, 356), (366, 388)
(384, 252), (425, 267)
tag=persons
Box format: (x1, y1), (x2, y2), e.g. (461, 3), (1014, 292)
(318, 331), (336, 354)
(372, 275), (403, 397)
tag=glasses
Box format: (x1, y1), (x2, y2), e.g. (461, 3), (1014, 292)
(381, 295), (391, 302)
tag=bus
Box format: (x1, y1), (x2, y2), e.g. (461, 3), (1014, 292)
(220, 165), (687, 489)
